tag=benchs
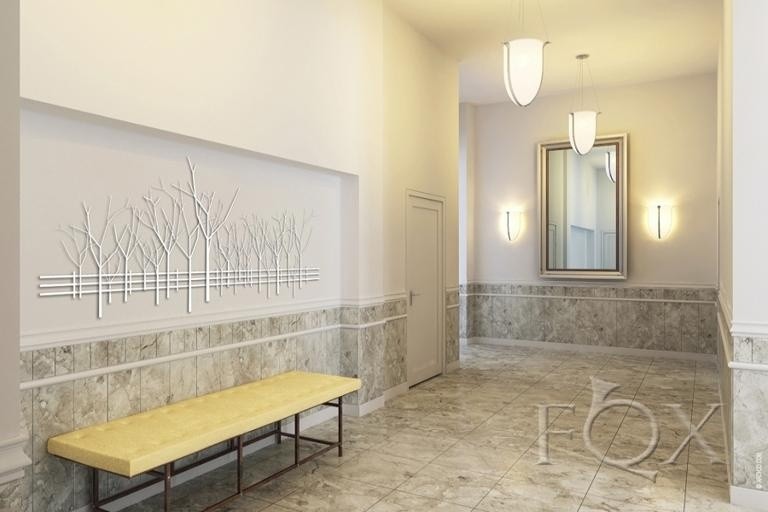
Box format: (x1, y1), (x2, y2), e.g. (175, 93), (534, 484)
(45, 369), (362, 511)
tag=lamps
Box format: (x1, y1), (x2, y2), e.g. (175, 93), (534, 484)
(646, 202), (673, 243)
(564, 50), (602, 160)
(500, 0), (554, 112)
(503, 207), (524, 246)
(603, 146), (618, 185)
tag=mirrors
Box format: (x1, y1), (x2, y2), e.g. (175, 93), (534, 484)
(536, 132), (629, 282)
